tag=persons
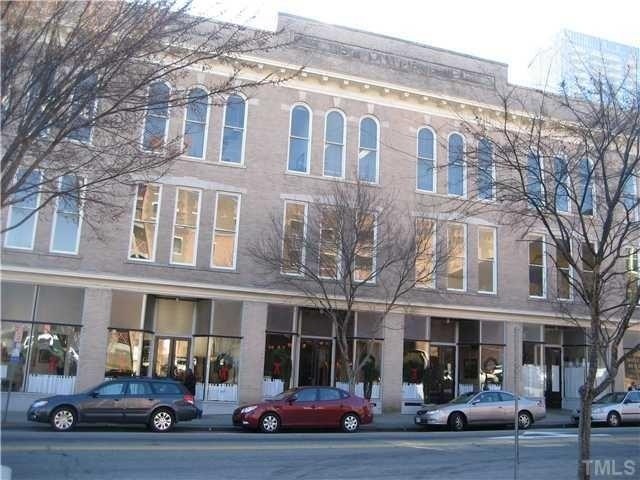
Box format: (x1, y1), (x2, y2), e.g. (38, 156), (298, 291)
(628, 380), (637, 391)
(182, 368), (196, 398)
(226, 364), (233, 384)
(218, 361), (229, 381)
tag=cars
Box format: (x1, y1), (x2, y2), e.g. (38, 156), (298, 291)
(481, 363), (505, 386)
(172, 356), (195, 376)
(572, 390), (640, 428)
(23, 374), (203, 432)
(230, 384), (376, 433)
(413, 387), (546, 431)
